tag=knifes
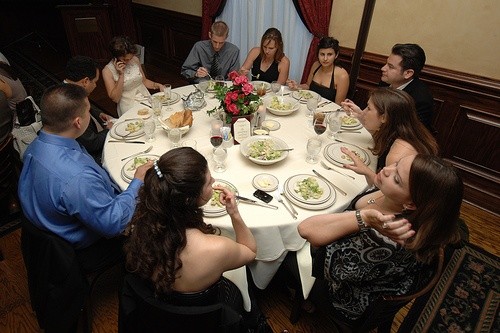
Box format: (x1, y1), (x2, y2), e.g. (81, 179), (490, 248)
(312, 170), (346, 196)
(108, 140), (145, 144)
(235, 195), (278, 210)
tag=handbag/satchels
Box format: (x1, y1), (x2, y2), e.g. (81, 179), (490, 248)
(11, 94), (43, 163)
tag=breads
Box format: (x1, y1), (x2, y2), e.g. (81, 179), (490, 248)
(166, 110), (193, 128)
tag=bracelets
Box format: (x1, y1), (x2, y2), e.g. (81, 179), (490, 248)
(154, 82), (159, 89)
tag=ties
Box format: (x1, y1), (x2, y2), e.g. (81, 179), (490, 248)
(211, 51), (220, 79)
(90, 113), (104, 133)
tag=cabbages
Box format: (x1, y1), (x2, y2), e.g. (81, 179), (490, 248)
(270, 97), (293, 111)
(298, 91), (312, 98)
(342, 116), (357, 124)
(127, 157), (151, 171)
(126, 122), (143, 130)
(209, 189), (224, 207)
(246, 141), (282, 160)
(293, 176), (323, 200)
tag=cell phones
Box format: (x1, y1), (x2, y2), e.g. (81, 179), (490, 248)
(253, 189), (273, 203)
(112, 56), (121, 63)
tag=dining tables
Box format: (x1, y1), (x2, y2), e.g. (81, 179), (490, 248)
(102, 81), (378, 312)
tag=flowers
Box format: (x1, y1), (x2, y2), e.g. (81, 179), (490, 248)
(208, 72), (263, 124)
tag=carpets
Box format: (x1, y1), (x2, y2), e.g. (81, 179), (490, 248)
(395, 243), (500, 333)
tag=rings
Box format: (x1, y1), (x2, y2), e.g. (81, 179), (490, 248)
(382, 223), (387, 229)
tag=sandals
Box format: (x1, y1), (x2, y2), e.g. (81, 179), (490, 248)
(289, 295), (315, 313)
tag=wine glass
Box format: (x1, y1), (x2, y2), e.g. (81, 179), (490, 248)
(151, 96), (163, 119)
(199, 80), (208, 101)
(163, 83), (173, 111)
(142, 119), (156, 143)
(209, 119), (227, 173)
(305, 136), (322, 165)
(305, 97), (318, 118)
(167, 128), (181, 149)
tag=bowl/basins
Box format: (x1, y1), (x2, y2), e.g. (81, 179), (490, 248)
(240, 135), (289, 166)
(252, 126), (271, 135)
(263, 96), (301, 115)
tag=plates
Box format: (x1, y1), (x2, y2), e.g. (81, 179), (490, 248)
(323, 142), (370, 168)
(200, 179), (239, 217)
(110, 81), (363, 140)
(253, 173), (279, 192)
(283, 174), (337, 211)
(121, 154), (160, 184)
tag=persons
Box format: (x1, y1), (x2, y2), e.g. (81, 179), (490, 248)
(63, 52), (118, 159)
(341, 43), (436, 138)
(239, 28), (290, 86)
(285, 36), (349, 106)
(102, 36), (164, 118)
(121, 147), (272, 333)
(181, 21), (240, 84)
(285, 154), (463, 328)
(359, 88), (437, 174)
(18, 82), (153, 272)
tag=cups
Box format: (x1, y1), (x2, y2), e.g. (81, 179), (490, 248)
(256, 83), (266, 99)
(215, 75), (224, 87)
(271, 80), (281, 94)
(182, 139), (198, 150)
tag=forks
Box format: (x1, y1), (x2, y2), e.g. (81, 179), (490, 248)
(320, 161), (355, 180)
(121, 146), (153, 161)
(248, 71), (257, 77)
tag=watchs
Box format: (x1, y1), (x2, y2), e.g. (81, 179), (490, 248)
(356, 210), (371, 233)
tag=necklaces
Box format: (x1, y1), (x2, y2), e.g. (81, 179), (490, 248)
(367, 195), (386, 206)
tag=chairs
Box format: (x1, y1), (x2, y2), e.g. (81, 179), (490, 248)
(362, 241), (446, 333)
(20, 217), (123, 333)
(118, 271), (225, 333)
